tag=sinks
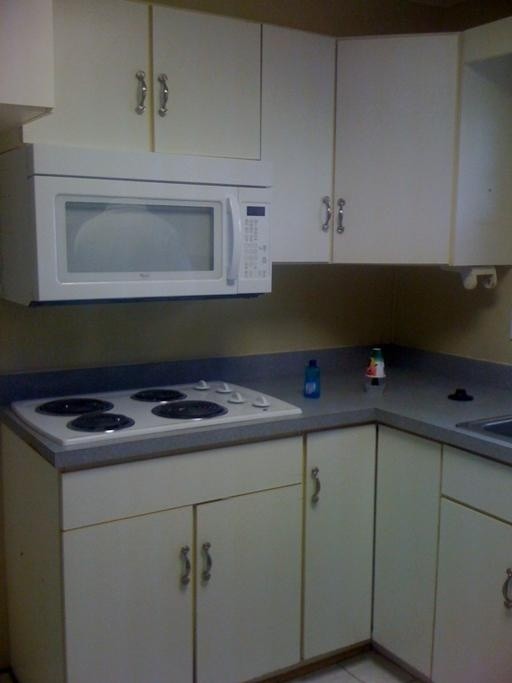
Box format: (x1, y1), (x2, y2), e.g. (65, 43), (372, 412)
(455, 415), (512, 444)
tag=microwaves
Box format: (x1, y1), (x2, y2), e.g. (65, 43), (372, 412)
(0, 142), (274, 309)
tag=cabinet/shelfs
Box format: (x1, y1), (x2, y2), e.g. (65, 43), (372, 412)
(58, 434), (303, 681)
(22, 0), (260, 159)
(307, 422), (380, 664)
(259, 24), (334, 267)
(381, 425), (512, 683)
(334, 16), (512, 266)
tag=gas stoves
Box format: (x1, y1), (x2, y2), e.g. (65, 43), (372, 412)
(8, 375), (301, 453)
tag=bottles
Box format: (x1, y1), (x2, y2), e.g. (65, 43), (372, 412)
(363, 346), (388, 378)
